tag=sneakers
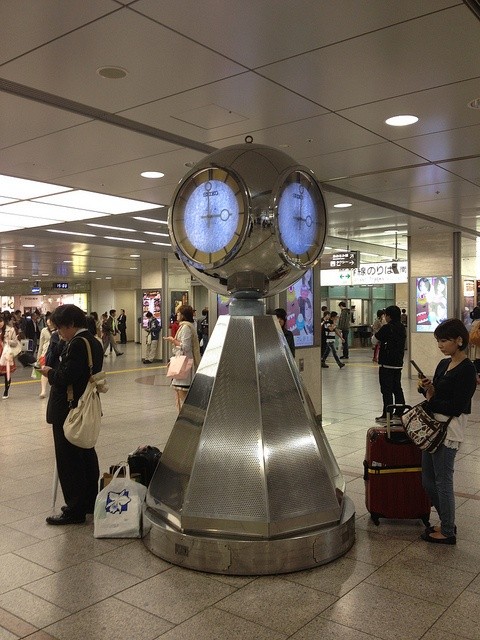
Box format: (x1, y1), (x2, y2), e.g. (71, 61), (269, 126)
(321, 363), (329, 368)
(375, 415), (393, 423)
(340, 364), (345, 368)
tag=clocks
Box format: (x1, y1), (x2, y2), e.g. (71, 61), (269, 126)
(276, 167), (326, 267)
(170, 166), (246, 264)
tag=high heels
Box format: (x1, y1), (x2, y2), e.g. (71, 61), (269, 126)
(2, 391), (10, 400)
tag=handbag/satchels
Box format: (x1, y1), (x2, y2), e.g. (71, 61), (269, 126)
(127, 443), (162, 484)
(399, 403), (447, 455)
(166, 349), (194, 381)
(90, 460), (146, 539)
(469, 322), (480, 345)
(63, 369), (109, 449)
(97, 455), (144, 494)
(10, 340), (22, 358)
(31, 361), (41, 380)
(109, 461), (126, 476)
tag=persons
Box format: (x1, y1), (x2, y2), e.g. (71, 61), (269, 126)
(197, 308), (209, 357)
(85, 315), (103, 349)
(192, 310), (197, 333)
(371, 306), (407, 421)
(422, 318), (477, 544)
(337, 302), (351, 360)
(171, 315), (179, 337)
(34, 304), (103, 525)
(89, 311), (102, 339)
(117, 309), (127, 344)
(321, 311), (331, 356)
(103, 310), (124, 357)
(10, 311), (29, 339)
(351, 305), (356, 322)
(321, 306), (328, 320)
(401, 308), (407, 351)
(273, 308), (295, 359)
(163, 305), (197, 414)
(101, 312), (112, 356)
(143, 312), (161, 364)
(0, 313), (18, 399)
(468, 307), (480, 383)
(322, 311), (346, 368)
(372, 310), (385, 363)
(33, 307), (45, 353)
(300, 269), (311, 299)
(300, 298), (313, 337)
(45, 332), (66, 370)
(36, 314), (58, 398)
(428, 277), (447, 323)
(27, 313), (40, 363)
(416, 277), (431, 324)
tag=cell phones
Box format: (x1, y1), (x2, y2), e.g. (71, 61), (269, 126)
(410, 360), (430, 381)
(27, 362), (46, 371)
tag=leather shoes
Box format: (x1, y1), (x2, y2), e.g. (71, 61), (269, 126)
(420, 530), (457, 545)
(61, 504), (94, 514)
(425, 525), (457, 534)
(46, 513), (86, 526)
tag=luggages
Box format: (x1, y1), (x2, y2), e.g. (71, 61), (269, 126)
(363, 404), (433, 528)
